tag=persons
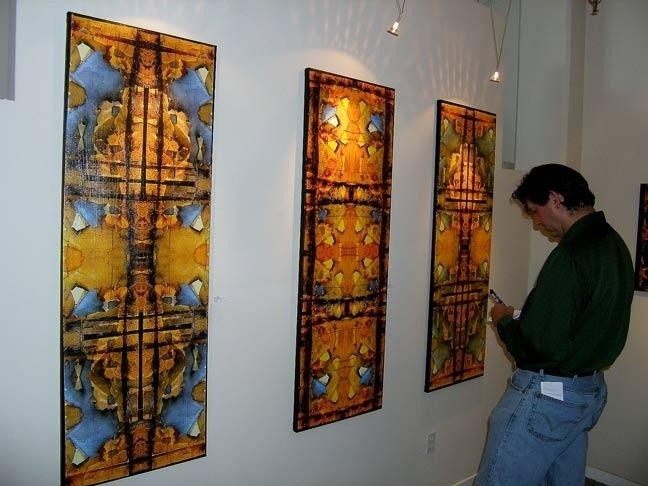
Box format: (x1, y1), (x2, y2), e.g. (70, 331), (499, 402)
(473, 161), (636, 485)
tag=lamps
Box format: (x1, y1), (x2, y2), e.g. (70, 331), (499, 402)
(480, 0), (513, 86)
(385, 0), (407, 37)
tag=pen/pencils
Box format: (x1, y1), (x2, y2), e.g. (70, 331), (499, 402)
(490, 289), (504, 304)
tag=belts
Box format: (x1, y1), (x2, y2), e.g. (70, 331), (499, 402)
(515, 361), (600, 378)
